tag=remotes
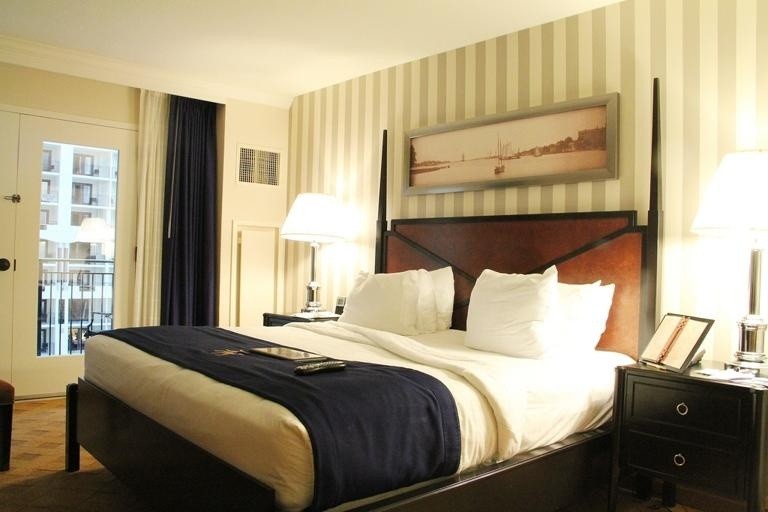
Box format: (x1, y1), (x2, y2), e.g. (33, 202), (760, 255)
(295, 360), (347, 375)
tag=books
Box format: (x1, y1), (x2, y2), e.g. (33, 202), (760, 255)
(638, 311), (715, 373)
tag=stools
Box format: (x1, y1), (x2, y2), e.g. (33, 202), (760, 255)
(0, 380), (15, 472)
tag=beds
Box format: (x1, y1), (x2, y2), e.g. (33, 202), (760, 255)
(64, 77), (661, 511)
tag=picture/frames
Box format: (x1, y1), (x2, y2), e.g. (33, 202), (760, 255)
(402, 91), (620, 196)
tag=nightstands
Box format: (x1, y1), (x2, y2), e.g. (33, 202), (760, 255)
(260, 312), (341, 327)
(612, 363), (767, 512)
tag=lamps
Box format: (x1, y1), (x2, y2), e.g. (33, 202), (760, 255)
(684, 148), (767, 376)
(281, 192), (348, 316)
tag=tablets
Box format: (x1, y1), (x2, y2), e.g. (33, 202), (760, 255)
(247, 346), (328, 361)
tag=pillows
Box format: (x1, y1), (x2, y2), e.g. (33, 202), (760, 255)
(335, 268), (438, 335)
(427, 265), (456, 331)
(463, 265), (617, 371)
